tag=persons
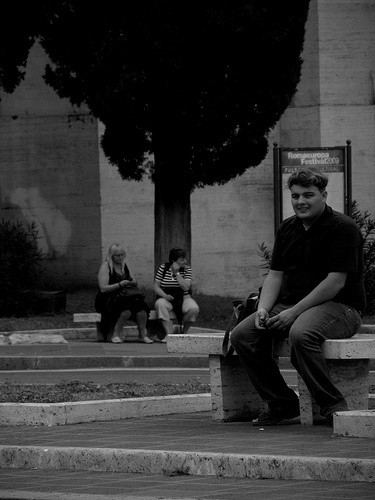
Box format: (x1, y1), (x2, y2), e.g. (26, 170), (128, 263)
(153, 247), (200, 342)
(230, 167), (367, 428)
(95, 243), (154, 344)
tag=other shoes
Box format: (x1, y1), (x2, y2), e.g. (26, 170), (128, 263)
(111, 336), (122, 343)
(252, 411), (301, 427)
(136, 335), (154, 344)
(161, 334), (167, 342)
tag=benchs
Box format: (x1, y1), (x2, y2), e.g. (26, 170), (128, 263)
(167, 332), (375, 427)
(73, 311), (177, 342)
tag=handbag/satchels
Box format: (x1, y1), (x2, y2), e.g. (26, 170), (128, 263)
(222, 286), (262, 358)
(120, 286), (145, 302)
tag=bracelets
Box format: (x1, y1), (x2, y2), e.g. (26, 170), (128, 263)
(119, 282), (122, 288)
(173, 272), (180, 278)
